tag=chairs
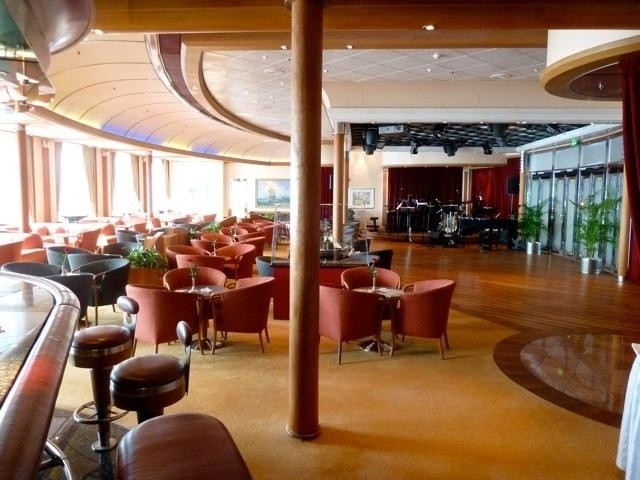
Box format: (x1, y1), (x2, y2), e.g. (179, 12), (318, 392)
(152, 227), (190, 257)
(46, 245), (93, 271)
(23, 233), (43, 248)
(166, 245), (204, 267)
(243, 216), (276, 243)
(71, 258), (131, 322)
(212, 244), (257, 278)
(56, 227), (65, 233)
(107, 237), (118, 244)
(0, 261), (94, 332)
(107, 320), (192, 480)
(369, 250), (393, 268)
(173, 215), (193, 222)
(66, 253), (123, 271)
(354, 239), (370, 251)
(70, 296), (137, 479)
(115, 219), (125, 225)
(320, 284), (382, 364)
(126, 284), (204, 358)
(117, 230), (140, 243)
(74, 228), (101, 254)
(135, 223), (149, 232)
(39, 227), (49, 235)
(21, 249), (47, 263)
(341, 266), (401, 289)
(390, 279), (456, 360)
(240, 231), (264, 241)
(163, 265), (226, 292)
(102, 224), (115, 235)
(254, 256), (288, 276)
(104, 242), (143, 258)
(212, 277), (277, 353)
(174, 255), (225, 273)
(152, 218), (161, 228)
(203, 214), (216, 222)
(219, 226), (248, 241)
(238, 236), (266, 264)
(0, 240), (25, 264)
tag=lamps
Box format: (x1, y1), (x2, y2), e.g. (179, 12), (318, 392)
(443, 141), (457, 156)
(534, 69), (538, 71)
(427, 68), (431, 71)
(489, 73), (512, 81)
(362, 127), (377, 155)
(482, 142), (492, 154)
(280, 45), (287, 49)
(409, 138), (420, 154)
(599, 82), (604, 90)
(424, 25), (435, 31)
(433, 53), (440, 59)
(347, 45), (352, 49)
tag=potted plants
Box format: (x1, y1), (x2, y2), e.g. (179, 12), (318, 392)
(534, 203), (548, 254)
(127, 249), (169, 288)
(589, 196), (622, 273)
(568, 194), (598, 274)
(519, 204), (533, 254)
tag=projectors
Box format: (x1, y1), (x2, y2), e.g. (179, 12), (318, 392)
(378, 124), (411, 135)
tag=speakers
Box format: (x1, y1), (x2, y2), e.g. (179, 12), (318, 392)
(506, 175), (520, 194)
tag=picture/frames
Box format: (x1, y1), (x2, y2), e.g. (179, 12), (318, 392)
(255, 179), (291, 208)
(348, 187), (374, 209)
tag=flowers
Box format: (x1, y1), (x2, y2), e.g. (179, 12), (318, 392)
(187, 258), (202, 277)
(368, 258), (380, 277)
(61, 246), (72, 265)
(202, 232), (225, 247)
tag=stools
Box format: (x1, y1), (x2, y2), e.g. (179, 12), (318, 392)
(369, 217), (381, 232)
(117, 412), (253, 479)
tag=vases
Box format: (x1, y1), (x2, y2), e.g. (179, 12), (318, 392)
(190, 278), (196, 293)
(373, 277), (376, 289)
(214, 248), (217, 256)
(61, 265), (65, 277)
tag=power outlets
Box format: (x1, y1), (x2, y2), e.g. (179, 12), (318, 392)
(242, 178), (247, 184)
(237, 177), (240, 183)
(228, 176), (235, 183)
